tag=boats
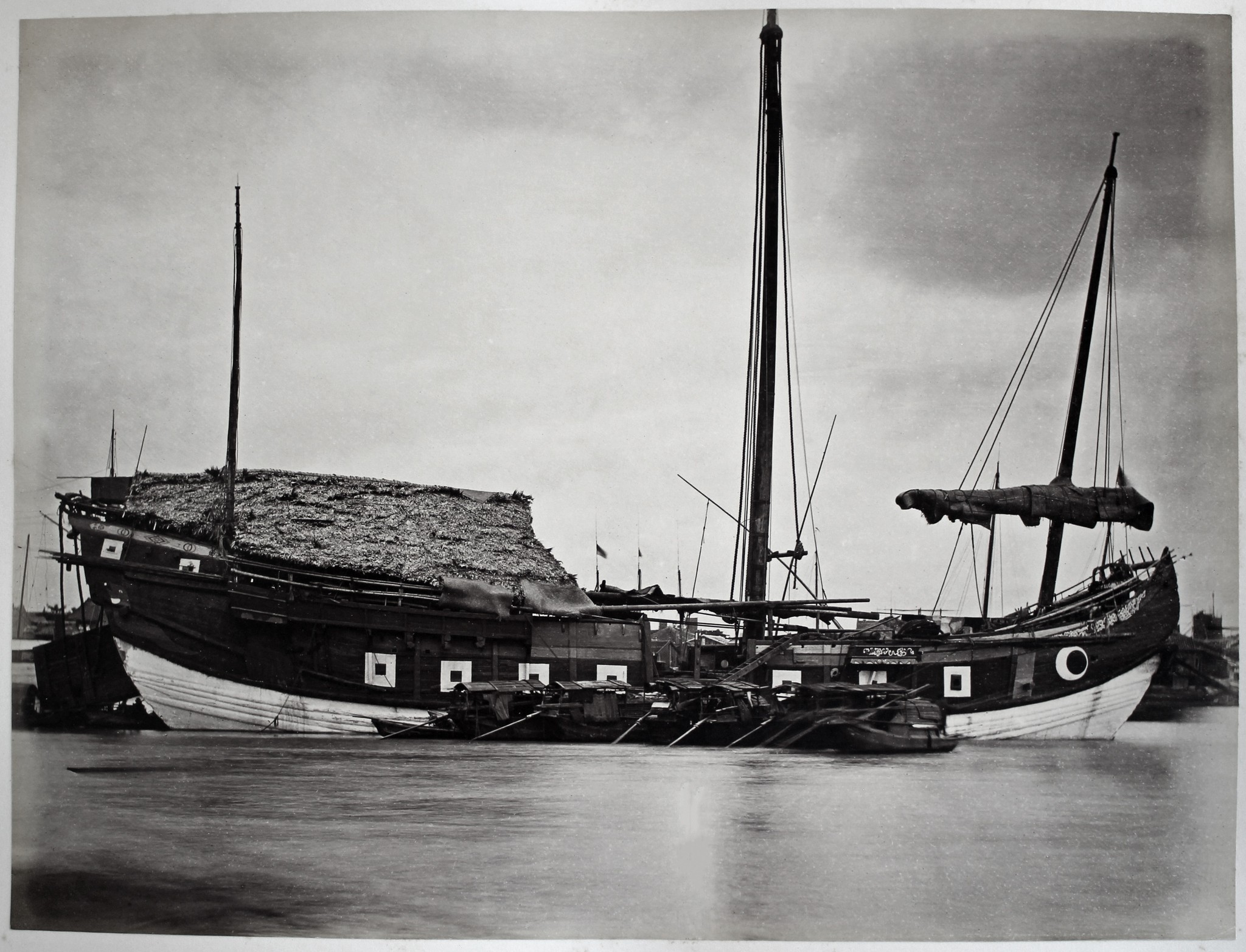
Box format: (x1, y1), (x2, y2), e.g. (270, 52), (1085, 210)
(367, 674), (977, 757)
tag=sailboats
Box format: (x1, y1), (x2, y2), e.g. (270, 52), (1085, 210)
(41, 13), (1186, 755)
